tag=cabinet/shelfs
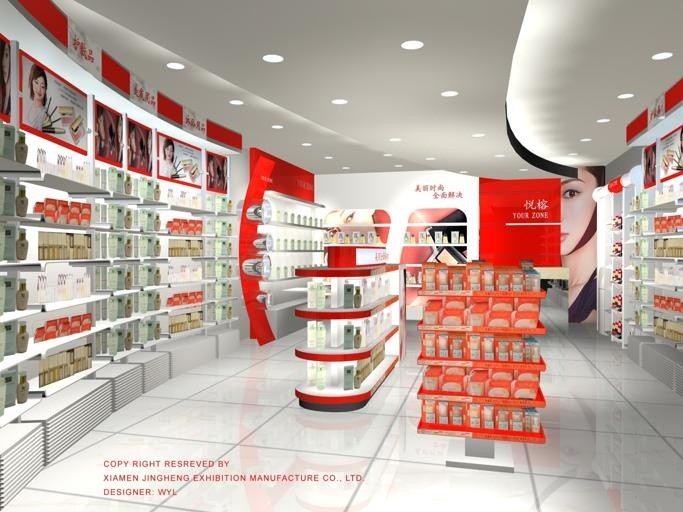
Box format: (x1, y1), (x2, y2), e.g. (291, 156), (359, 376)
(596, 176), (683, 353)
(0, 36), (547, 509)
